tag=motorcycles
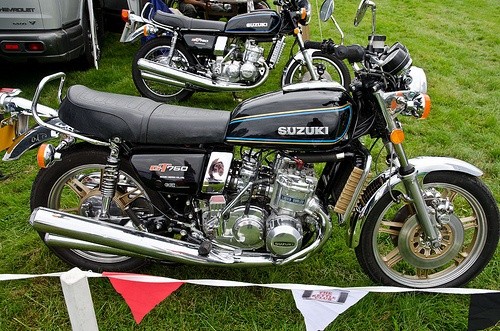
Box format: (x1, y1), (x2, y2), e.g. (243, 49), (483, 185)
(119, 0), (351, 106)
(0, 0), (500, 297)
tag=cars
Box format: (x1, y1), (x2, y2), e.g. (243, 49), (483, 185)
(0, 0), (107, 76)
